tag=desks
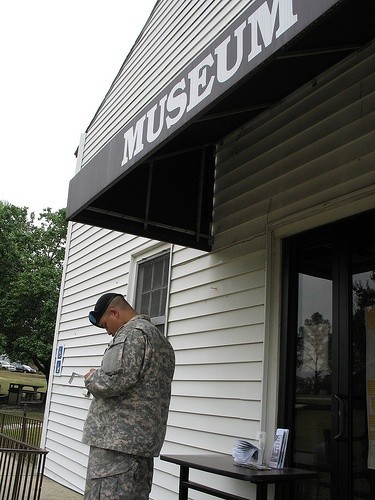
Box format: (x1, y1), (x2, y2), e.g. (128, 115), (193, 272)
(160, 455), (319, 500)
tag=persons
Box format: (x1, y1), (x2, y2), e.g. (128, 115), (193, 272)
(81, 292), (175, 499)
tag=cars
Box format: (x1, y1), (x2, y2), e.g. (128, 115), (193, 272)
(15, 365), (37, 374)
(0, 360), (17, 372)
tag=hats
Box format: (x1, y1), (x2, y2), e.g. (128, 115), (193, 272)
(88, 293), (124, 327)
